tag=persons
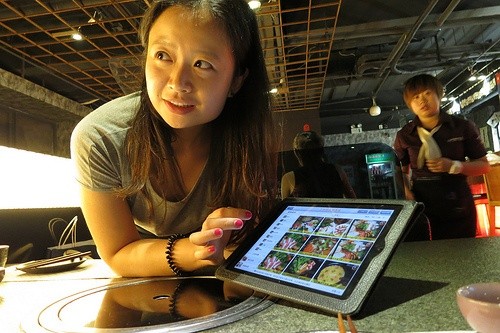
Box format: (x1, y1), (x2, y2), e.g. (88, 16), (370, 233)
(70, 1), (276, 278)
(105, 283), (220, 324)
(281, 130), (356, 201)
(393, 73), (490, 240)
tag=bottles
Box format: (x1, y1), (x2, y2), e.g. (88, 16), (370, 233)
(369, 164), (396, 199)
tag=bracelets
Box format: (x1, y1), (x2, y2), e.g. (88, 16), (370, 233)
(164, 232), (189, 277)
(169, 282), (186, 320)
(449, 160), (463, 175)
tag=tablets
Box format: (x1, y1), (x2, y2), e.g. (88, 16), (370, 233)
(214, 193), (421, 316)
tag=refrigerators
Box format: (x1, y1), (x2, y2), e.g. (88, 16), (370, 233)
(366, 151), (402, 201)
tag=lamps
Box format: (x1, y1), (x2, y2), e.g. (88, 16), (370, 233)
(370, 96), (381, 116)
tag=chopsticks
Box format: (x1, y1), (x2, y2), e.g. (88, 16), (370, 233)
(26, 251), (92, 268)
(338, 312), (358, 333)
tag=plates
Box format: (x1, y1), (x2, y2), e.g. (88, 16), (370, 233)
(0, 266), (9, 284)
(16, 256), (87, 274)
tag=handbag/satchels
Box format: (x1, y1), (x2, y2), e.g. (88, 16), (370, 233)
(47, 216), (98, 258)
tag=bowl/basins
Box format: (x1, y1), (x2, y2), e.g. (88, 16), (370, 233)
(0, 244), (10, 266)
(456, 282), (500, 333)
